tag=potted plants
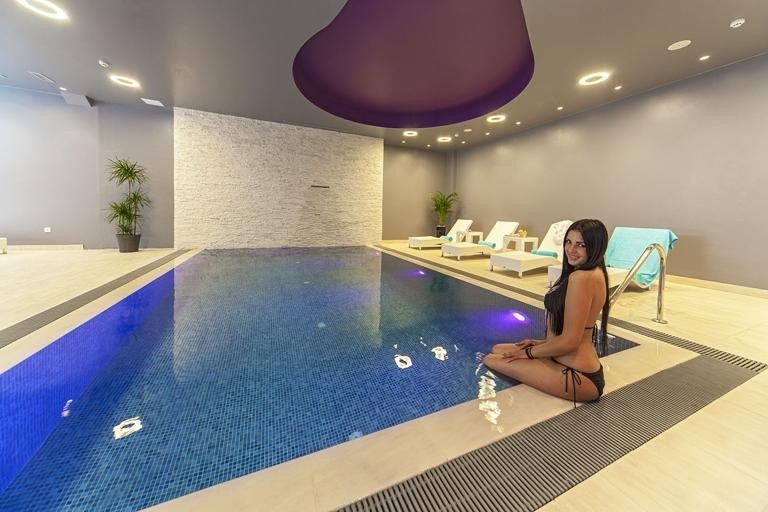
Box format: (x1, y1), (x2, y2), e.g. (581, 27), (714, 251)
(102, 156), (152, 251)
(429, 190), (457, 236)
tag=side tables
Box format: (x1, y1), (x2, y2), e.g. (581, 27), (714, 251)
(456, 231), (483, 243)
(503, 235), (539, 254)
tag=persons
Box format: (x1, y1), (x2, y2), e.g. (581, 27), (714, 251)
(482, 219), (609, 403)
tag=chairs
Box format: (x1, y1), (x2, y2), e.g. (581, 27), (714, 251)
(491, 221), (575, 278)
(548, 228), (676, 295)
(441, 219), (520, 260)
(409, 219), (474, 251)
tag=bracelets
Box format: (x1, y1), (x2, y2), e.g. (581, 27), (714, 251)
(525, 347), (532, 359)
(529, 347), (534, 359)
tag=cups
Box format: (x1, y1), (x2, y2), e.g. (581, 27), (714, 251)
(519, 232), (527, 238)
